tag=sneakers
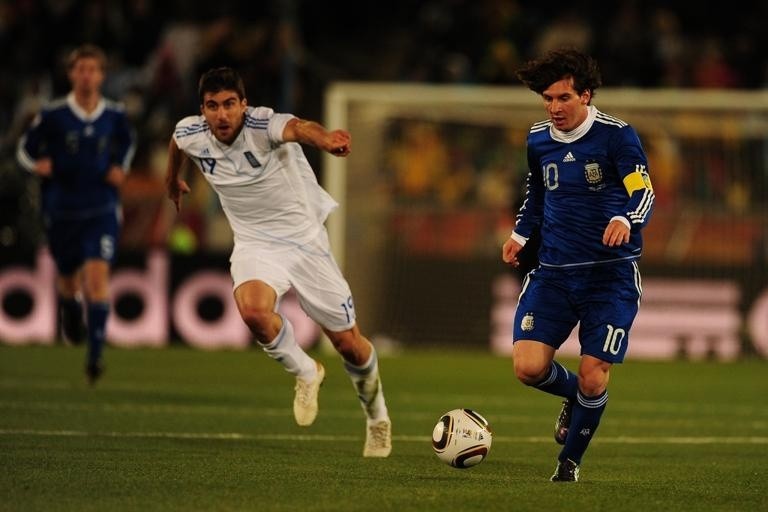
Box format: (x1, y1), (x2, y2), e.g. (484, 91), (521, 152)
(549, 459), (581, 483)
(83, 342), (105, 385)
(362, 418), (394, 458)
(553, 398), (575, 446)
(61, 294), (89, 348)
(292, 359), (326, 426)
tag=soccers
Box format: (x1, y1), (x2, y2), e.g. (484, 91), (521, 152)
(432, 408), (492, 468)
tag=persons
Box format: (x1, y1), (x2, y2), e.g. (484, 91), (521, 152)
(501, 47), (656, 483)
(2, 0), (766, 273)
(161, 65), (393, 459)
(15, 44), (140, 386)
